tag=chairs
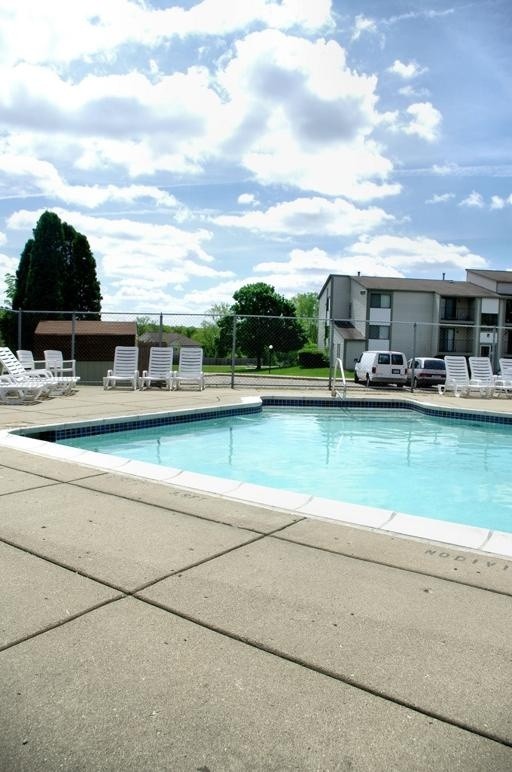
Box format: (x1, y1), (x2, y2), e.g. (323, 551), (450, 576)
(431, 354), (512, 399)
(0, 347), (206, 403)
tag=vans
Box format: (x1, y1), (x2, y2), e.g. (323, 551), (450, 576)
(406, 357), (446, 388)
(353, 351), (408, 388)
